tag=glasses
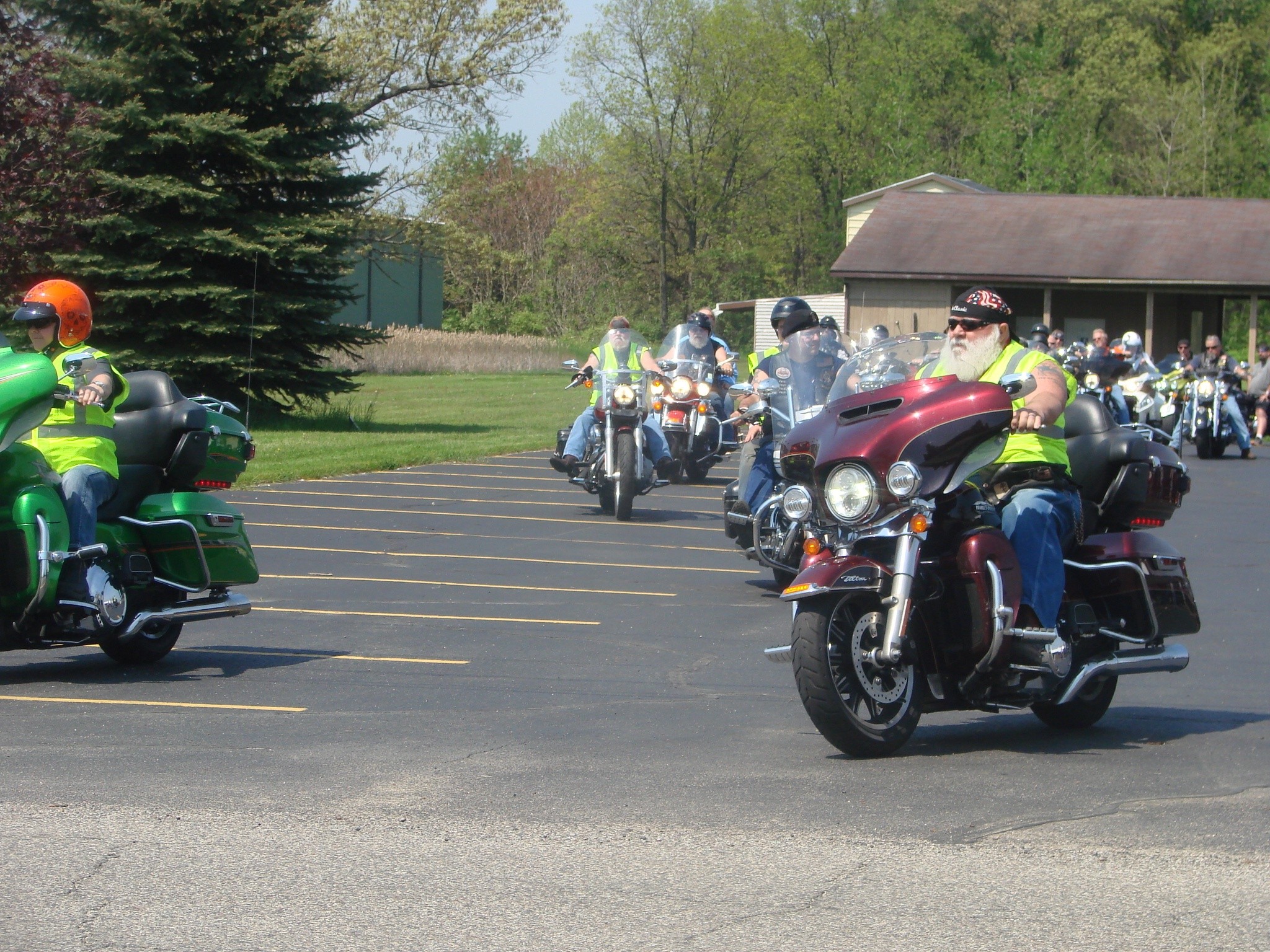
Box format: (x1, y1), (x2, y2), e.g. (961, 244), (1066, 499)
(1179, 346), (1189, 349)
(25, 318), (55, 329)
(1205, 346), (1217, 350)
(611, 329), (629, 334)
(1093, 337), (1101, 341)
(822, 325), (835, 329)
(947, 318), (990, 331)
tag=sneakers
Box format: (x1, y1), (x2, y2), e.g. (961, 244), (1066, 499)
(56, 558), (88, 601)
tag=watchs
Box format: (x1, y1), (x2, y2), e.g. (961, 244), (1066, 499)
(90, 381), (106, 395)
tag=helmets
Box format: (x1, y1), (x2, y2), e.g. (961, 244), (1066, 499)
(869, 325), (889, 339)
(608, 316), (630, 329)
(1122, 331), (1142, 345)
(687, 313), (711, 331)
(1032, 323), (1049, 333)
(820, 316), (836, 327)
(1018, 337), (1028, 348)
(771, 296), (813, 329)
(1032, 335), (1047, 344)
(12, 279), (92, 349)
(1052, 330), (1064, 339)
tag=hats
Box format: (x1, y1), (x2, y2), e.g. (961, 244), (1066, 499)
(950, 284), (1014, 324)
(782, 309), (820, 338)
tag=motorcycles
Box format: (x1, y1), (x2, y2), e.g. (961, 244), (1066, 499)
(0, 333), (259, 665)
(764, 332), (1201, 759)
(719, 329), (941, 589)
(1026, 337), (1270, 461)
(555, 328), (678, 522)
(652, 323), (743, 485)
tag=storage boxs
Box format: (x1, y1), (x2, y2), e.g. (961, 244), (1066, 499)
(1085, 531), (1202, 636)
(1145, 443), (1191, 515)
(195, 409), (256, 484)
(142, 492), (259, 586)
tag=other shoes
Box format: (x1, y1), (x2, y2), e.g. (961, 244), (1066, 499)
(1251, 437), (1262, 446)
(730, 499), (755, 548)
(657, 458), (681, 484)
(1169, 446), (1178, 455)
(1241, 450), (1256, 459)
(550, 454), (580, 477)
(1012, 638), (1045, 666)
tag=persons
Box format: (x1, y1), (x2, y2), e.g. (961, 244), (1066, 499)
(11, 279), (128, 601)
(1087, 328), (1130, 429)
(1242, 341), (1270, 447)
(819, 316), (850, 362)
(653, 306), (740, 455)
(1019, 323), (1064, 354)
(913, 284), (1083, 694)
(1111, 331), (1159, 380)
(730, 309), (864, 549)
(738, 296), (813, 504)
(1155, 338), (1198, 402)
(859, 325), (906, 374)
(549, 315), (682, 480)
(1168, 334), (1257, 459)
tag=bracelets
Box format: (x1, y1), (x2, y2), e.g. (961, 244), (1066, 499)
(1265, 390), (1270, 396)
